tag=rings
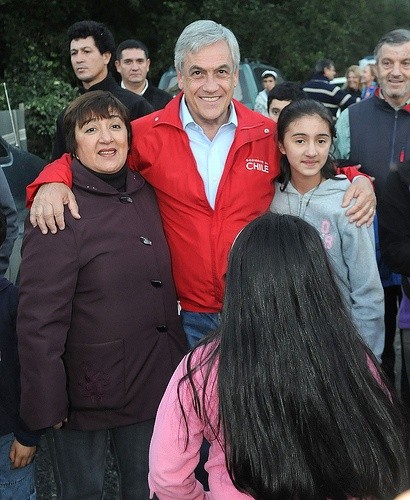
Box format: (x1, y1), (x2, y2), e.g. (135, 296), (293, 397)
(370, 209), (375, 214)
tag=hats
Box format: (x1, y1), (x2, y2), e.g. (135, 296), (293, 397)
(261, 70), (278, 79)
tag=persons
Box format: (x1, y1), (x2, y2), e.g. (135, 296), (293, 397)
(265, 97), (387, 369)
(267, 82), (309, 123)
(329, 28), (410, 416)
(344, 65), (366, 94)
(359, 64), (380, 102)
(23, 19), (378, 351)
(253, 70), (279, 119)
(303, 57), (363, 124)
(50, 20), (156, 163)
(115, 39), (175, 112)
(146, 211), (409, 500)
(0, 166), (19, 278)
(16, 90), (193, 500)
(0, 277), (44, 500)
